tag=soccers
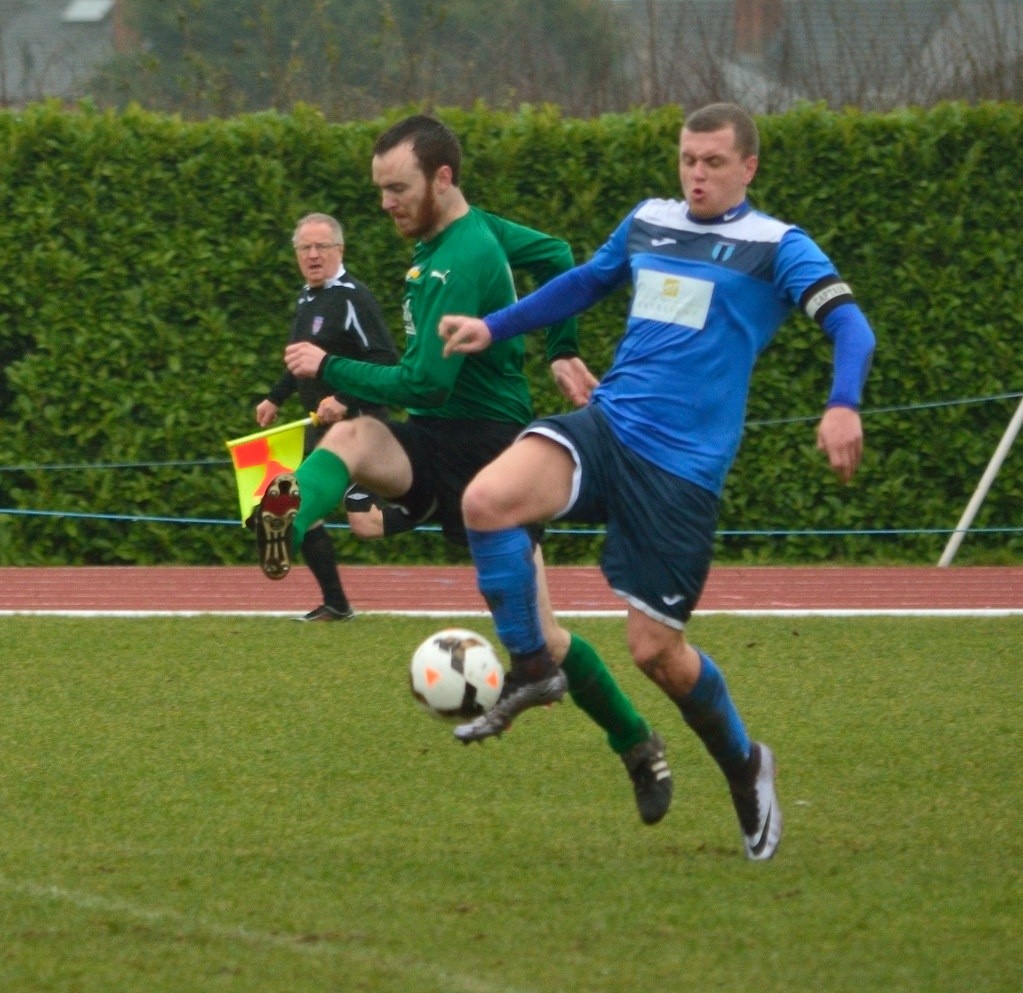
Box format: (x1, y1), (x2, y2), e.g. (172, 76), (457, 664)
(410, 628), (507, 720)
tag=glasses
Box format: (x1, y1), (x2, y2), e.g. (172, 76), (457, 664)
(293, 243), (339, 252)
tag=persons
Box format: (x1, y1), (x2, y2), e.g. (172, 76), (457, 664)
(437, 101), (876, 859)
(254, 212), (418, 625)
(285, 115), (674, 827)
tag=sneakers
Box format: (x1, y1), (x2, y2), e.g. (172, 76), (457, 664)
(257, 473), (300, 581)
(290, 603), (353, 625)
(451, 668), (568, 742)
(731, 741), (781, 860)
(620, 729), (671, 825)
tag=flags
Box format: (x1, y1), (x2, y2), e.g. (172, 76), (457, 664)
(226, 420), (305, 527)
